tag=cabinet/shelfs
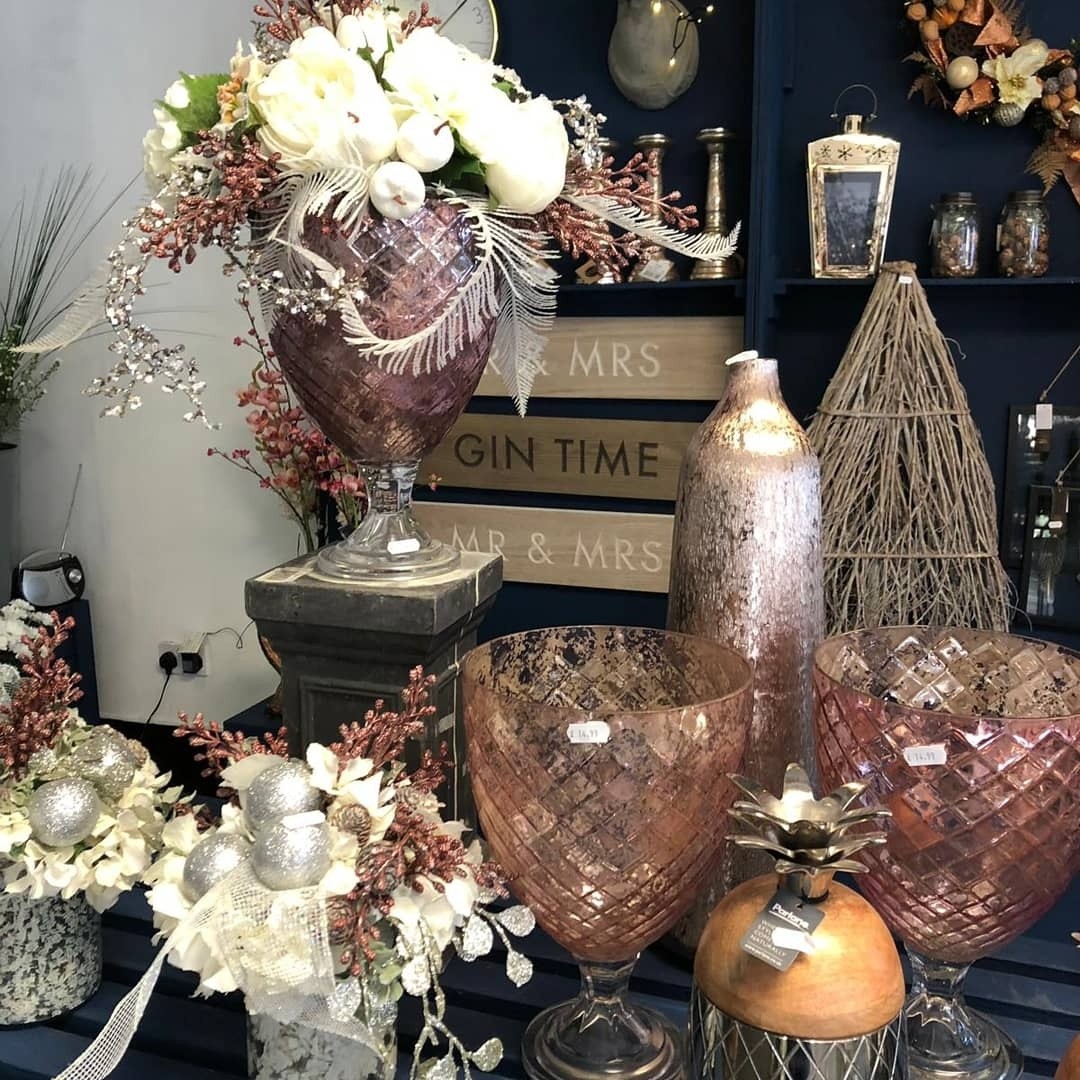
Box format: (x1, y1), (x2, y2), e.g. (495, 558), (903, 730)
(494, 0), (1080, 655)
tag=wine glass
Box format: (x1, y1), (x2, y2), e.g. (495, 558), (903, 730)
(811, 625), (1080, 1080)
(460, 626), (754, 1080)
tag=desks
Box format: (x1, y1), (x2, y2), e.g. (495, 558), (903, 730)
(1, 797), (1080, 1080)
(244, 552), (504, 848)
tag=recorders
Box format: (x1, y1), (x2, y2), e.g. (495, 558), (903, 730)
(12, 464), (86, 611)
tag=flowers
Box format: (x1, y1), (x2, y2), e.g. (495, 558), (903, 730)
(131, 662), (535, 1080)
(0, 599), (184, 911)
(900, 0), (1080, 204)
(6, 0), (742, 522)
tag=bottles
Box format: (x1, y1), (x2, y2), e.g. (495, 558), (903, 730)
(931, 192), (981, 278)
(995, 190), (1051, 279)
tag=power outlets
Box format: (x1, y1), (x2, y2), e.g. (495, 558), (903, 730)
(159, 641), (208, 677)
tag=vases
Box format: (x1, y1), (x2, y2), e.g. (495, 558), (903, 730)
(0, 859), (102, 1027)
(246, 1010), (397, 1080)
(252, 200), (497, 581)
